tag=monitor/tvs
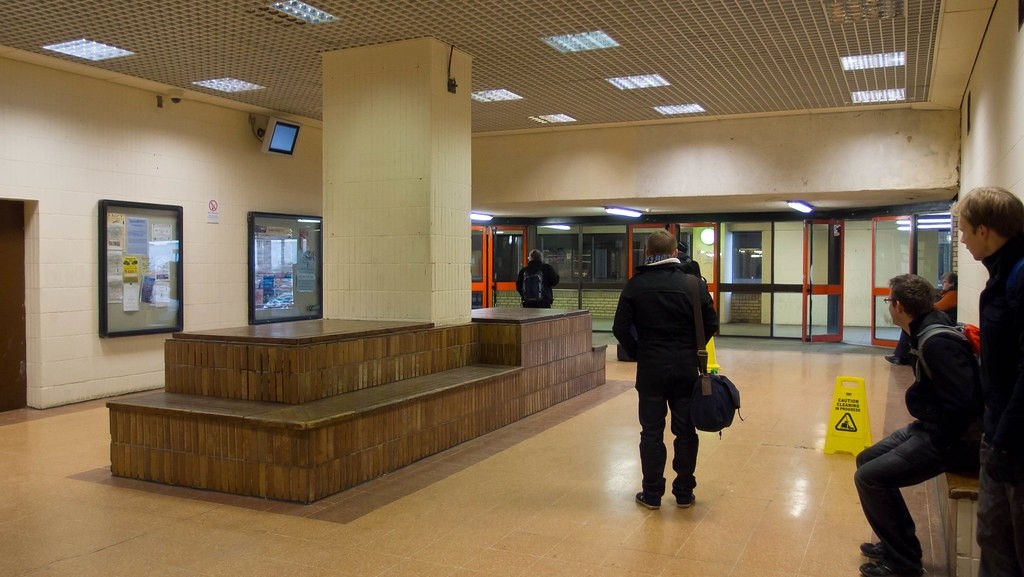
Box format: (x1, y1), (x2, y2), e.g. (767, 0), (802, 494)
(261, 116), (301, 157)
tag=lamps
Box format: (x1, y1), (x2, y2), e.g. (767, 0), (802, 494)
(786, 200), (813, 213)
(471, 213), (493, 222)
(605, 206), (642, 218)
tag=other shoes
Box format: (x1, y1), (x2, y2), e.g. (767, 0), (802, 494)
(860, 560), (903, 577)
(636, 492), (661, 510)
(884, 354), (902, 365)
(861, 542), (887, 558)
(676, 494), (695, 507)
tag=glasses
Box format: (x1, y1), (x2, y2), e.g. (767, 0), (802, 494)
(884, 297), (906, 310)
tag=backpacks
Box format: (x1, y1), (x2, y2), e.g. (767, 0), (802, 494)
(917, 319), (981, 358)
(519, 262), (548, 303)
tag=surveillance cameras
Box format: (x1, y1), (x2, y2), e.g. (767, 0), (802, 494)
(169, 90), (182, 103)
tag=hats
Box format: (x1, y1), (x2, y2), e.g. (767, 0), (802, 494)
(677, 241), (688, 253)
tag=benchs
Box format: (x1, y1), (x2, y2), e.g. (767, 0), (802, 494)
(106, 343), (608, 507)
(937, 468), (982, 577)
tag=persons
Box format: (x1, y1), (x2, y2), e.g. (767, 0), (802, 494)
(678, 241), (700, 276)
(612, 228), (719, 510)
(516, 250), (560, 309)
(884, 272), (958, 366)
(853, 273), (983, 577)
(953, 184), (1024, 577)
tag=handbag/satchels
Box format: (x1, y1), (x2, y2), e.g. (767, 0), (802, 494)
(689, 372), (739, 432)
